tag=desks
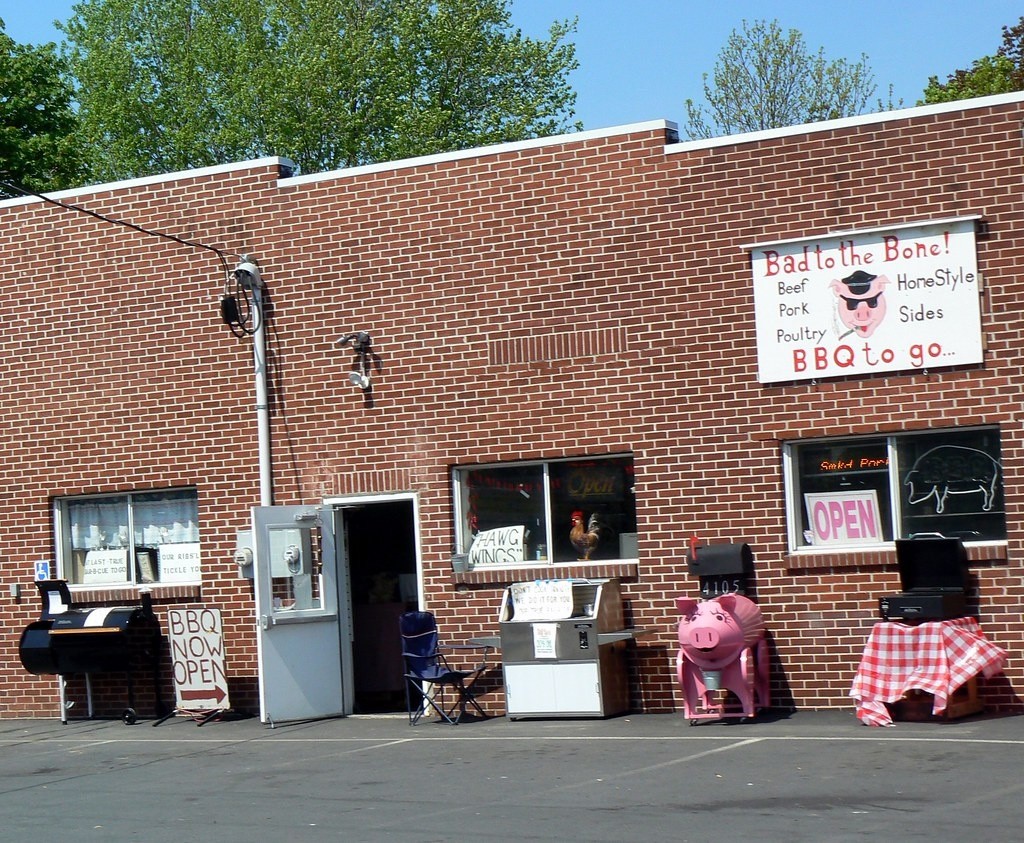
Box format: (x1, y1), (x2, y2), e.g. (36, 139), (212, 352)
(875, 617), (979, 720)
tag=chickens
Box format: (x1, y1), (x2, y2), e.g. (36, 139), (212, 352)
(569, 511), (600, 561)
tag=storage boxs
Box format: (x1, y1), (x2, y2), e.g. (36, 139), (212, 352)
(878, 532), (969, 621)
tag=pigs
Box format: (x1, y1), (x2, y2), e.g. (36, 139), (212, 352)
(672, 592), (765, 669)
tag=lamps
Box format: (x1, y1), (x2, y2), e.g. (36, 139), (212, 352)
(336, 330), (370, 390)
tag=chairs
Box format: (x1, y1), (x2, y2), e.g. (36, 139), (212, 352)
(399, 611), (489, 725)
